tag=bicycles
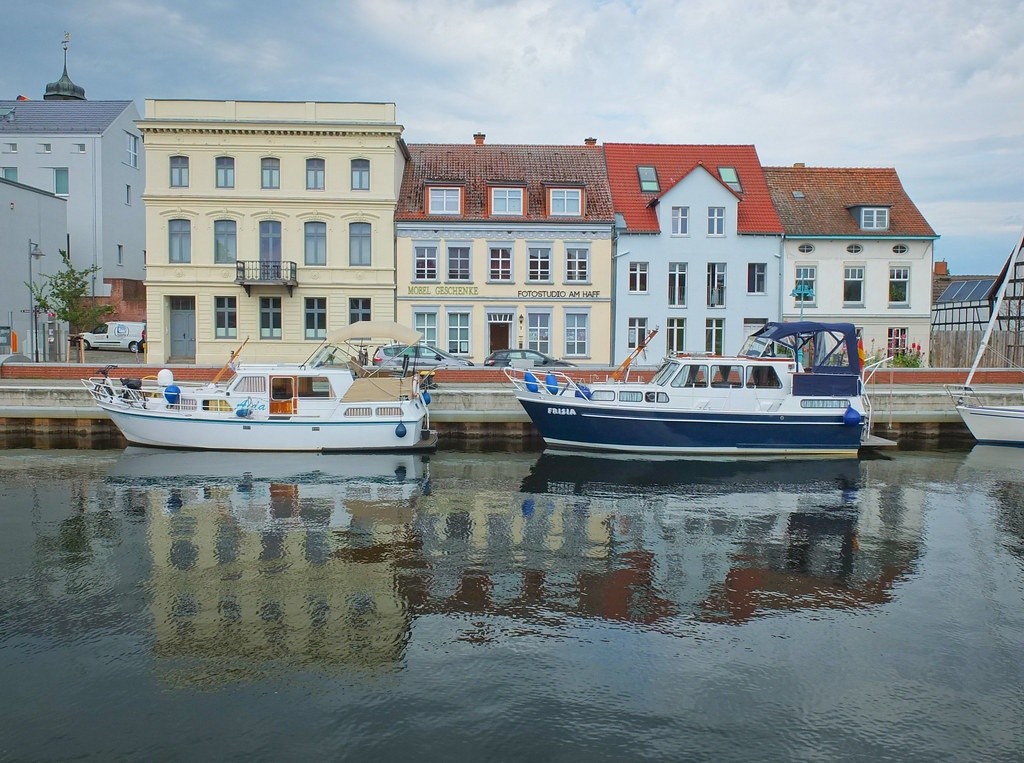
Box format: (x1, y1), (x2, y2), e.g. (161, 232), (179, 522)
(354, 343), (368, 365)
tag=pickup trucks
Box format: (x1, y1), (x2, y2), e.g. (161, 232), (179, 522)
(72, 322), (146, 353)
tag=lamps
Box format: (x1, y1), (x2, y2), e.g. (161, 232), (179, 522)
(519, 314), (524, 324)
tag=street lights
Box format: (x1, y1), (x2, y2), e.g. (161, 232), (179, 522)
(28, 238), (46, 362)
(789, 271), (815, 361)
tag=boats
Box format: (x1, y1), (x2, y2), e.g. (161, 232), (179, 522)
(82, 320), (436, 452)
(503, 322), (865, 455)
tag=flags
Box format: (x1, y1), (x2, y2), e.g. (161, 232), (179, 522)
(859, 336), (864, 369)
(16, 94), (31, 100)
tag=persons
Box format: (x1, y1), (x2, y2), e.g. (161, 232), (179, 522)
(841, 344), (848, 363)
(142, 324), (147, 342)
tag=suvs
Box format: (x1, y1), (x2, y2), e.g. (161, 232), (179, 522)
(372, 343), (475, 367)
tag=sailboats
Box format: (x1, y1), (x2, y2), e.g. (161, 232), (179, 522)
(943, 218), (1024, 447)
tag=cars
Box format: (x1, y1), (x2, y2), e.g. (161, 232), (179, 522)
(484, 349), (578, 367)
